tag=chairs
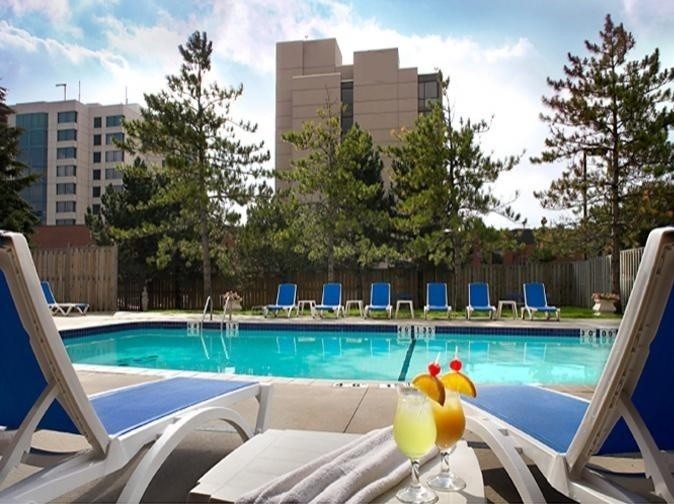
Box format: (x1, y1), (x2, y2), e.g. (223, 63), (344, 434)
(41, 281), (88, 316)
(364, 282), (393, 319)
(264, 284), (298, 317)
(520, 281), (560, 321)
(423, 282), (452, 320)
(313, 283), (344, 319)
(466, 282), (497, 320)
(0, 229), (273, 504)
(461, 227), (674, 504)
(278, 336), (548, 366)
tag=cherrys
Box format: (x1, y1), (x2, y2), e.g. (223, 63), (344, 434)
(450, 359), (462, 371)
(428, 363), (440, 376)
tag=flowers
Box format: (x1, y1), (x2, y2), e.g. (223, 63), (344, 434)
(592, 292), (620, 301)
(224, 291), (241, 301)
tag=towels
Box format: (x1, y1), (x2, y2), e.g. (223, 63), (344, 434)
(234, 425), (439, 504)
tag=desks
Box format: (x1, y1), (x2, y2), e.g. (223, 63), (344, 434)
(297, 300), (316, 319)
(395, 300), (414, 320)
(346, 300), (363, 318)
(497, 300), (518, 320)
(184, 429), (484, 504)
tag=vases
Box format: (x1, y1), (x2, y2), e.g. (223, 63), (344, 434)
(223, 301), (242, 310)
(592, 300), (617, 312)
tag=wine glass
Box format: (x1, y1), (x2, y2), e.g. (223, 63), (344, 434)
(393, 385), (467, 501)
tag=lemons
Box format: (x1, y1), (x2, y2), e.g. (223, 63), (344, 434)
(440, 372), (477, 398)
(412, 374), (445, 406)
